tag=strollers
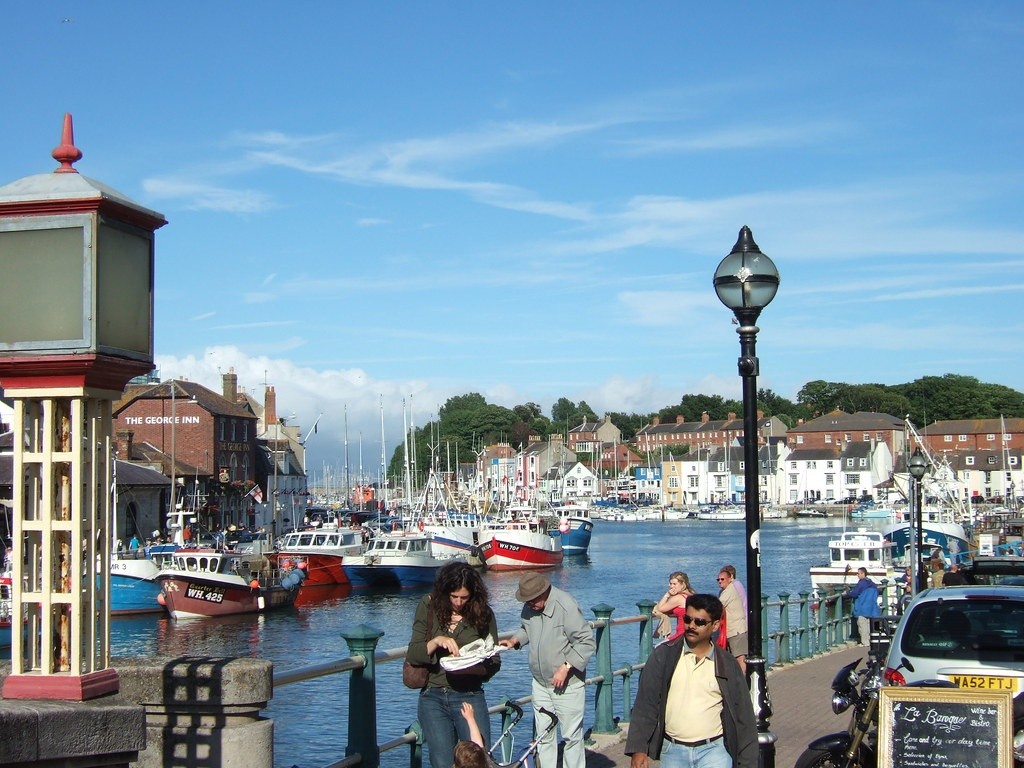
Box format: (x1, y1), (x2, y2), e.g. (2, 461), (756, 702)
(486, 700), (559, 768)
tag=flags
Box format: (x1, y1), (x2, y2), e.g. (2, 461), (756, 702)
(250, 485), (263, 504)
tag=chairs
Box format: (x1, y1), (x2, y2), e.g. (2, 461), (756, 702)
(939, 611), (972, 640)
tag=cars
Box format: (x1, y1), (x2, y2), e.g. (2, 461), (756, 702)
(987, 495), (1004, 504)
(794, 497), (819, 505)
(882, 584), (1024, 702)
(972, 496), (984, 504)
(834, 497), (857, 504)
(813, 496), (841, 505)
(856, 494), (872, 504)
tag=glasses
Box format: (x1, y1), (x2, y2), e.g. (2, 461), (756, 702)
(682, 614), (717, 626)
(716, 578), (724, 581)
(523, 594), (543, 606)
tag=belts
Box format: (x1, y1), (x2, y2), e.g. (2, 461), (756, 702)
(664, 731), (724, 747)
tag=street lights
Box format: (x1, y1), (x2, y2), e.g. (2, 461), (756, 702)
(273, 415), (297, 520)
(710, 223), (783, 768)
(1010, 480), (1015, 519)
(907, 446), (929, 593)
(169, 384), (200, 544)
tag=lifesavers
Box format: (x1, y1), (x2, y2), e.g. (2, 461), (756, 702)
(418, 521), (424, 532)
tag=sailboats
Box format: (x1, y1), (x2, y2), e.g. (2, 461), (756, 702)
(0, 384), (1024, 650)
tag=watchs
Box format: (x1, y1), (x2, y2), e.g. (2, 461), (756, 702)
(564, 662), (571, 669)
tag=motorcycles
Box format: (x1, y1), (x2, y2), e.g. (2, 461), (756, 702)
(792, 647), (1024, 768)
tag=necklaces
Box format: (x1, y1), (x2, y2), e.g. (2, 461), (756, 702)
(452, 610), (461, 615)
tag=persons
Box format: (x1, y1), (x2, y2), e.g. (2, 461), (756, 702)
(716, 571), (749, 672)
(846, 567), (880, 647)
(896, 583), (912, 615)
(4, 534), (140, 571)
(720, 565), (748, 622)
(499, 574), (597, 768)
(624, 594), (759, 768)
(184, 524), (191, 544)
(453, 702), (500, 768)
(406, 563), (501, 768)
(900, 567), (920, 595)
(655, 572), (696, 648)
(917, 539), (978, 590)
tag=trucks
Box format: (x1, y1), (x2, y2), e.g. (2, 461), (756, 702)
(873, 492), (905, 505)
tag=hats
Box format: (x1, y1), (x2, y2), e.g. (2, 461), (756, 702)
(514, 573), (551, 602)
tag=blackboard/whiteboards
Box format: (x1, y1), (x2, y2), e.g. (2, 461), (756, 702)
(878, 687), (1013, 768)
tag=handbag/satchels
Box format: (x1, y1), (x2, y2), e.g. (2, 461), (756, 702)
(403, 593), (434, 689)
